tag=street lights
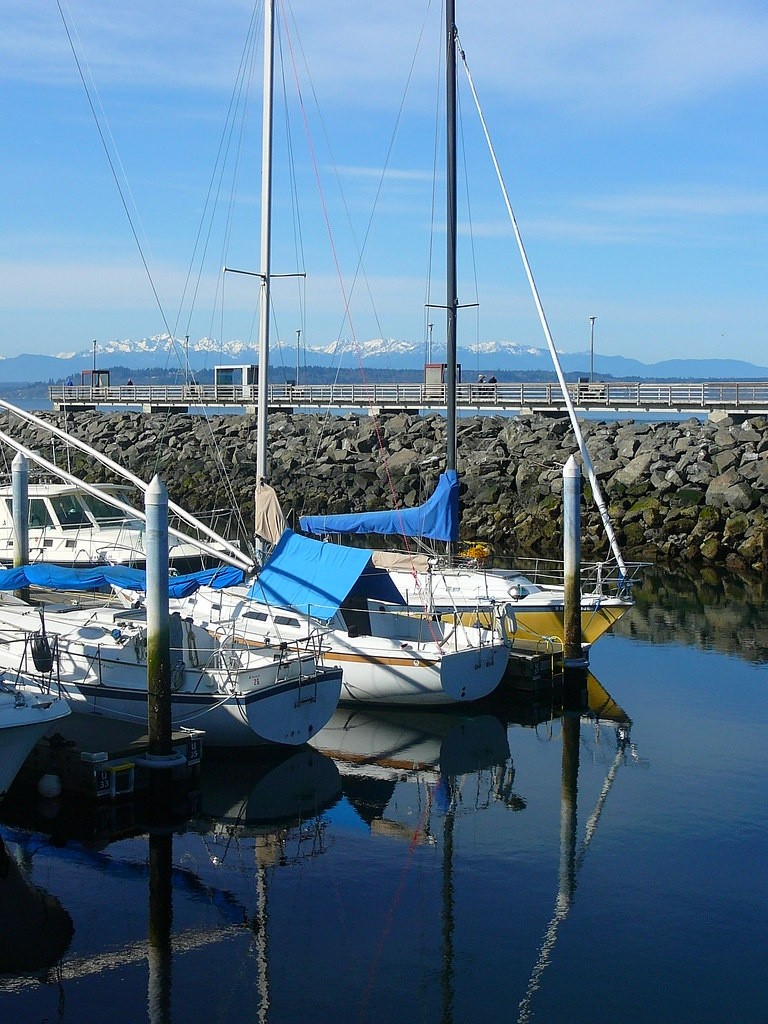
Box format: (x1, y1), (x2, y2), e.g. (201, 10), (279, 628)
(184, 334), (190, 386)
(91, 340), (97, 386)
(295, 330), (301, 388)
(428, 323), (434, 367)
(588, 315), (596, 381)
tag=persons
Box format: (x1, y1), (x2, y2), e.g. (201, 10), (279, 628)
(67, 378), (73, 397)
(478, 374), (497, 397)
(127, 379), (133, 397)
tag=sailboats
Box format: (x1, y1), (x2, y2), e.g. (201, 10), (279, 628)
(0, 1), (652, 796)
(174, 661), (631, 1024)
